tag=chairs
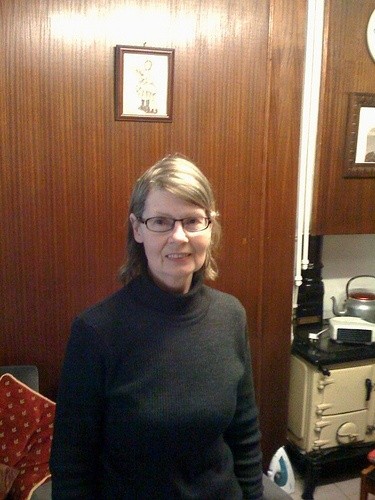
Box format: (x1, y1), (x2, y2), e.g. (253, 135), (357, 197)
(0, 365), (56, 500)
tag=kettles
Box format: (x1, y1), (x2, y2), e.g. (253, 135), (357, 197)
(330, 275), (375, 324)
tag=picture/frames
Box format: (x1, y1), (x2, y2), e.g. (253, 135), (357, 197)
(114, 44), (175, 124)
(343, 92), (375, 179)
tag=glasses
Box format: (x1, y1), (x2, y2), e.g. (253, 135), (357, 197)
(137, 214), (211, 232)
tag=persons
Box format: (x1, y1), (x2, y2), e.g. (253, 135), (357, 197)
(50, 157), (265, 500)
(136, 59), (156, 113)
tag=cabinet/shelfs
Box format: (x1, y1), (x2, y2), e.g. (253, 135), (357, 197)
(287, 353), (375, 500)
